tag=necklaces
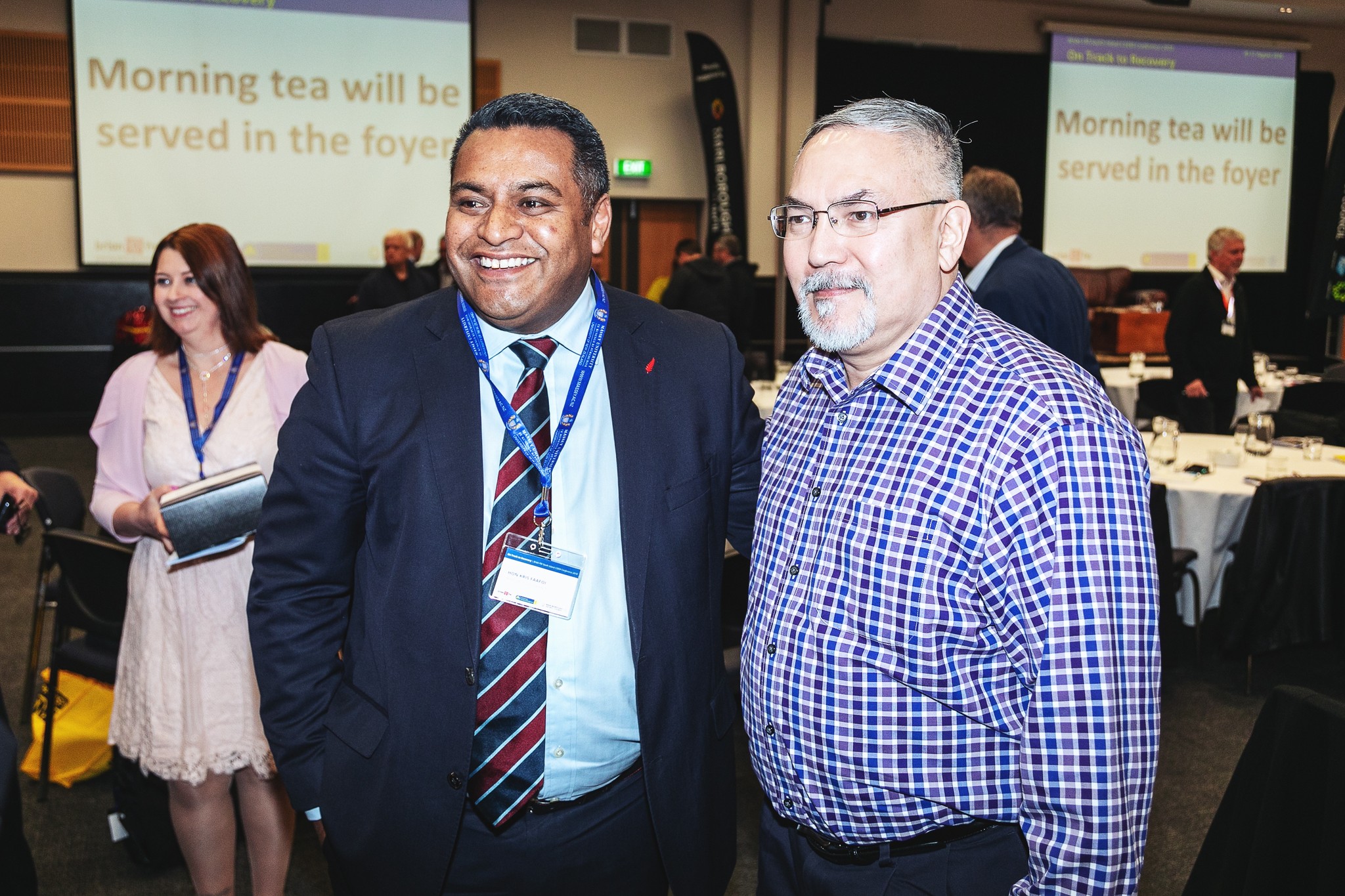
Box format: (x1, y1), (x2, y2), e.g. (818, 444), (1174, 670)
(180, 340), (232, 428)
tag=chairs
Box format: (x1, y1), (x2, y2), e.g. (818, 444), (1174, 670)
(1134, 364), (1345, 896)
(20, 469), (132, 800)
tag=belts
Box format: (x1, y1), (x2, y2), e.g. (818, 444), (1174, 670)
(766, 796), (998, 865)
(524, 760), (646, 816)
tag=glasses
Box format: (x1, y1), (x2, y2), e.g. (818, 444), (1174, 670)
(765, 200), (950, 238)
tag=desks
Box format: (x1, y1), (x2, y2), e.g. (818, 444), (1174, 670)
(1099, 366), (1323, 437)
(1130, 432), (1345, 630)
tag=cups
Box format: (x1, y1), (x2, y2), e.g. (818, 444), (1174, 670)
(1267, 362), (1298, 380)
(775, 359), (795, 387)
(1149, 415), (1180, 466)
(1244, 413), (1275, 456)
(1253, 351), (1265, 375)
(748, 380), (780, 417)
(1129, 351), (1146, 378)
(1303, 436), (1324, 460)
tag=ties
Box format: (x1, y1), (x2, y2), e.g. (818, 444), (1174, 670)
(470, 336), (552, 832)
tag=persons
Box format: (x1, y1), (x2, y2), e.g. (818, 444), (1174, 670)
(660, 236), (730, 329)
(736, 100), (1159, 895)
(89, 221), (313, 896)
(349, 229), (430, 311)
(960, 167), (1106, 391)
(405, 231), (424, 263)
(253, 98), (766, 896)
(1163, 226), (1263, 436)
(710, 233), (759, 376)
(0, 470), (38, 896)
(418, 235), (455, 297)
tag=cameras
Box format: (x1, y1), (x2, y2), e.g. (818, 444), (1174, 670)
(0, 493), (17, 534)
